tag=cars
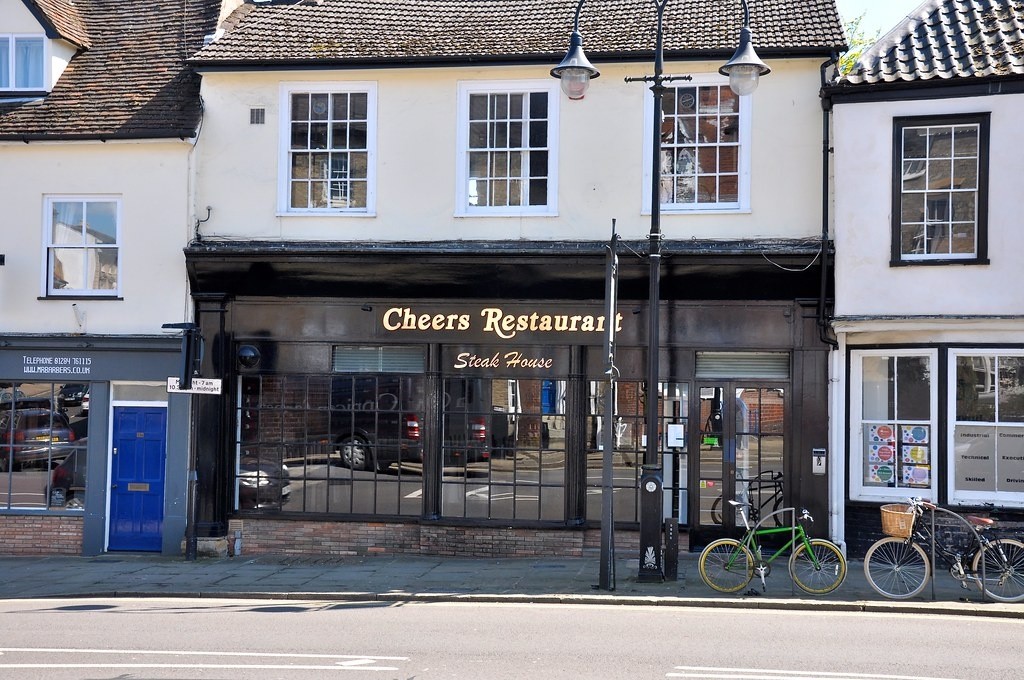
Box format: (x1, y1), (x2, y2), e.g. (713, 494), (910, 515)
(45, 438), (292, 512)
(56, 383), (89, 407)
(80, 389), (89, 413)
(0, 396), (55, 411)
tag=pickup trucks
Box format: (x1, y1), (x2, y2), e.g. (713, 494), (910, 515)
(324, 407), (490, 470)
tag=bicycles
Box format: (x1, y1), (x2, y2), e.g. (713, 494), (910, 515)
(697, 499), (848, 597)
(710, 471), (784, 526)
(864, 497), (1024, 609)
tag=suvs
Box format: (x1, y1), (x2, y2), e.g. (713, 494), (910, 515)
(0, 408), (76, 470)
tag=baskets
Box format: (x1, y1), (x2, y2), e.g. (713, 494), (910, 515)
(880, 504), (914, 538)
